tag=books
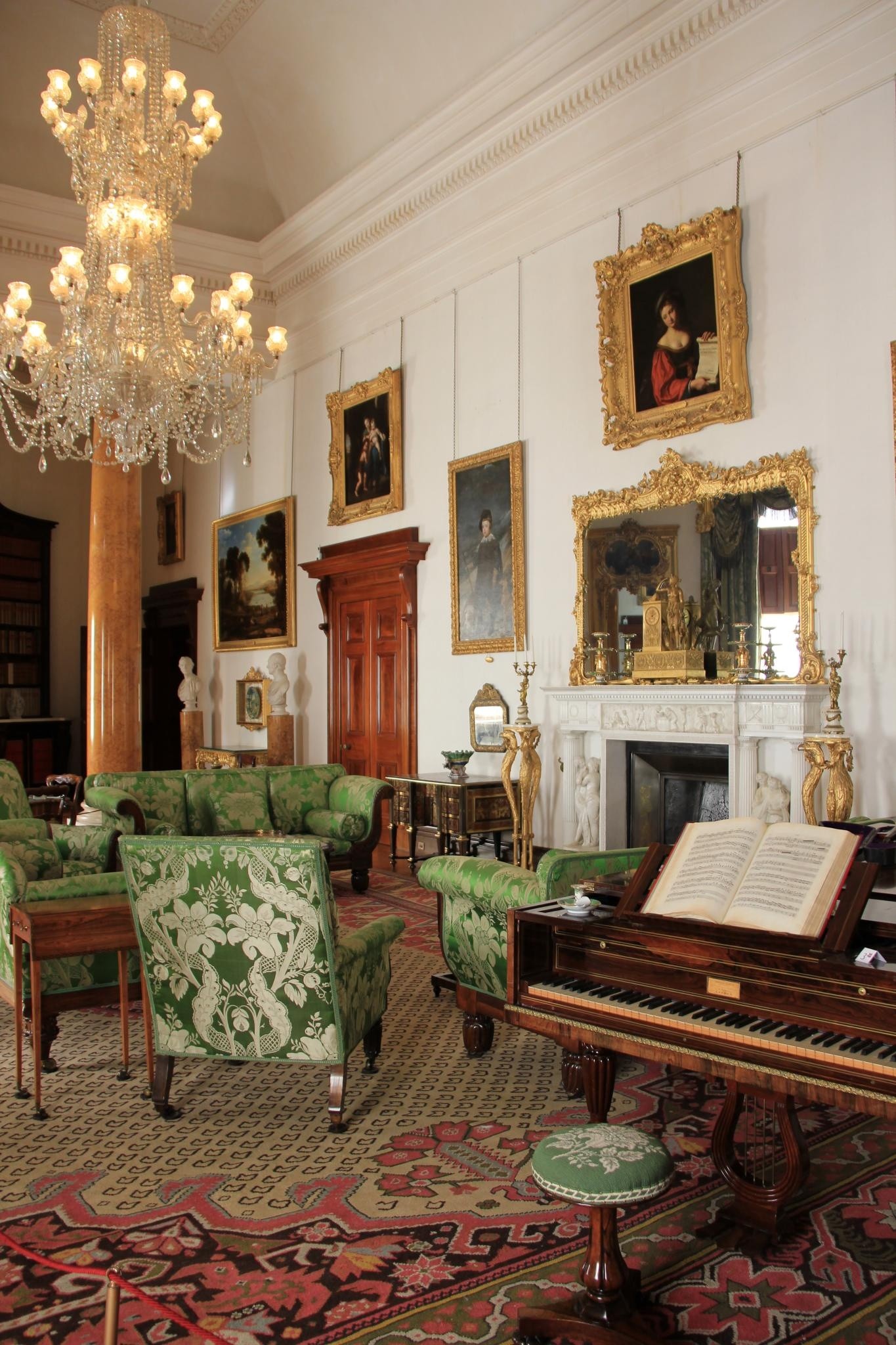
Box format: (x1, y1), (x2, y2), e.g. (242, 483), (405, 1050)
(0, 602), (41, 626)
(0, 630), (42, 655)
(0, 688), (41, 716)
(0, 662), (40, 684)
(640, 818), (864, 939)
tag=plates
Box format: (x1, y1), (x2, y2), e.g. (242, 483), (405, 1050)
(557, 898), (601, 913)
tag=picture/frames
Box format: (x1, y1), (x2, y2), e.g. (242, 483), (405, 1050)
(447, 439), (527, 652)
(592, 208), (753, 451)
(156, 490), (185, 565)
(211, 495), (297, 653)
(327, 364), (401, 527)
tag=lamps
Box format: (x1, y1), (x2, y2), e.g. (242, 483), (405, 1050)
(0, 0), (292, 479)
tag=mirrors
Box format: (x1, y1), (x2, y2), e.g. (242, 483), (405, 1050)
(565, 447), (825, 688)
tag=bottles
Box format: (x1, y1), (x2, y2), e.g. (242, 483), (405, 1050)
(6, 688), (24, 719)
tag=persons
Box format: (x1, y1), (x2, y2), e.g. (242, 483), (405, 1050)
(751, 771), (791, 824)
(572, 755), (600, 846)
(177, 657), (201, 711)
(266, 652), (289, 715)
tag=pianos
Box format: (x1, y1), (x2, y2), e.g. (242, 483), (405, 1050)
(499, 818), (896, 1242)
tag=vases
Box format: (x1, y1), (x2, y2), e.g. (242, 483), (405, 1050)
(7, 688), (26, 719)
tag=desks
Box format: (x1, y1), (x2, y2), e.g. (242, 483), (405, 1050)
(196, 746), (269, 769)
(9, 893), (155, 1120)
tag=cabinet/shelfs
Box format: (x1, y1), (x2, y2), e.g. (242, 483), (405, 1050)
(0, 502), (73, 786)
(385, 773), (524, 880)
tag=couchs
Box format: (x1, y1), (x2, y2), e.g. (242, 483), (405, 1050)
(82, 762), (398, 893)
(0, 759), (149, 1071)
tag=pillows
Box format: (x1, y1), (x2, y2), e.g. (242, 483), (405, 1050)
(205, 790), (273, 831)
(144, 817), (181, 836)
(303, 808), (364, 842)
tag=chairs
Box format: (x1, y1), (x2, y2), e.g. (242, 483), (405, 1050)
(27, 774), (81, 823)
(414, 844), (653, 1101)
(116, 830), (402, 1134)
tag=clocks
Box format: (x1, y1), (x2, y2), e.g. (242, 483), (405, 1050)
(631, 576), (709, 684)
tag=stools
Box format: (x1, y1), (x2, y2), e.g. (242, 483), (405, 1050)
(509, 1126), (683, 1345)
